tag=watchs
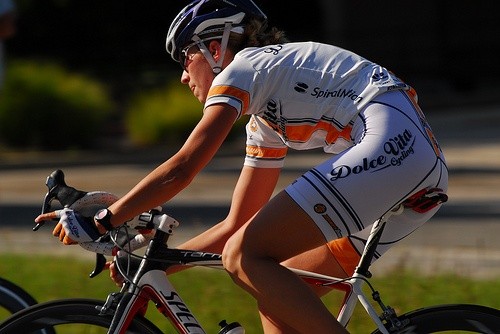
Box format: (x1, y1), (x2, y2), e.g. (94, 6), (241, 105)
(95, 208), (114, 232)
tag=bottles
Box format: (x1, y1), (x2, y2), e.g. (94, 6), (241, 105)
(217, 320), (246, 334)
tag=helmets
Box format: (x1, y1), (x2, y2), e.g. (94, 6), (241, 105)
(164, 0), (270, 61)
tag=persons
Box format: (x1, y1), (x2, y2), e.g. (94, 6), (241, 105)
(35, 0), (450, 334)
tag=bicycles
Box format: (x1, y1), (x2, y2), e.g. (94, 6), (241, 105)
(0, 168), (500, 333)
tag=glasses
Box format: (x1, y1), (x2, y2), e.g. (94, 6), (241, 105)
(179, 35), (225, 68)
